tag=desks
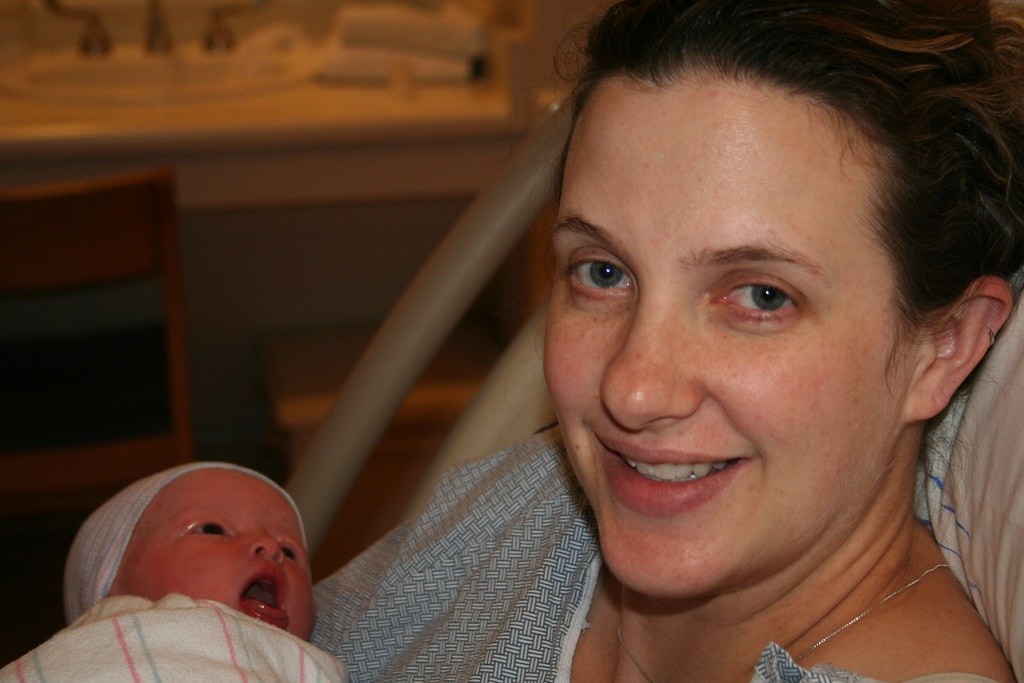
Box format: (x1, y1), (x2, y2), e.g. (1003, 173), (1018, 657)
(0, 78), (557, 447)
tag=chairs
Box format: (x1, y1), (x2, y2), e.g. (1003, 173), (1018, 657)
(0, 170), (198, 527)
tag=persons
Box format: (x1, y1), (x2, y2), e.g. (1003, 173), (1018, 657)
(311, 1), (1023, 683)
(0, 463), (349, 682)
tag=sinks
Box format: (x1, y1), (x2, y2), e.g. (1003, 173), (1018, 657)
(1, 43), (477, 99)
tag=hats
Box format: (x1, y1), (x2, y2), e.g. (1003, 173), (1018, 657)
(64, 462), (307, 628)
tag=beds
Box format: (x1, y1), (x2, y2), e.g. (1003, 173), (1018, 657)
(285, 96), (1024, 683)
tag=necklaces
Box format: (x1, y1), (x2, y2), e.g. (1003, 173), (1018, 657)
(618, 563), (953, 683)
